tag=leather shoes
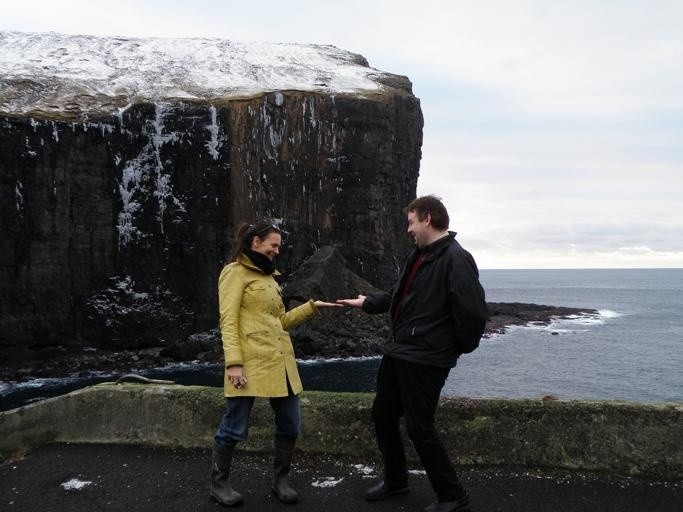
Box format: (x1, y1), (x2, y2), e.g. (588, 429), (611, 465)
(423, 489), (472, 512)
(364, 480), (410, 500)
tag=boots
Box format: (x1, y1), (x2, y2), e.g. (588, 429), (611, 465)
(272, 436), (298, 504)
(209, 438), (243, 507)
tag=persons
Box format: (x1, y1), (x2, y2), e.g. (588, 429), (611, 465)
(337, 196), (488, 511)
(205, 222), (343, 504)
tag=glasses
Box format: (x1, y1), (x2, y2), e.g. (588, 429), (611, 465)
(254, 224), (281, 235)
